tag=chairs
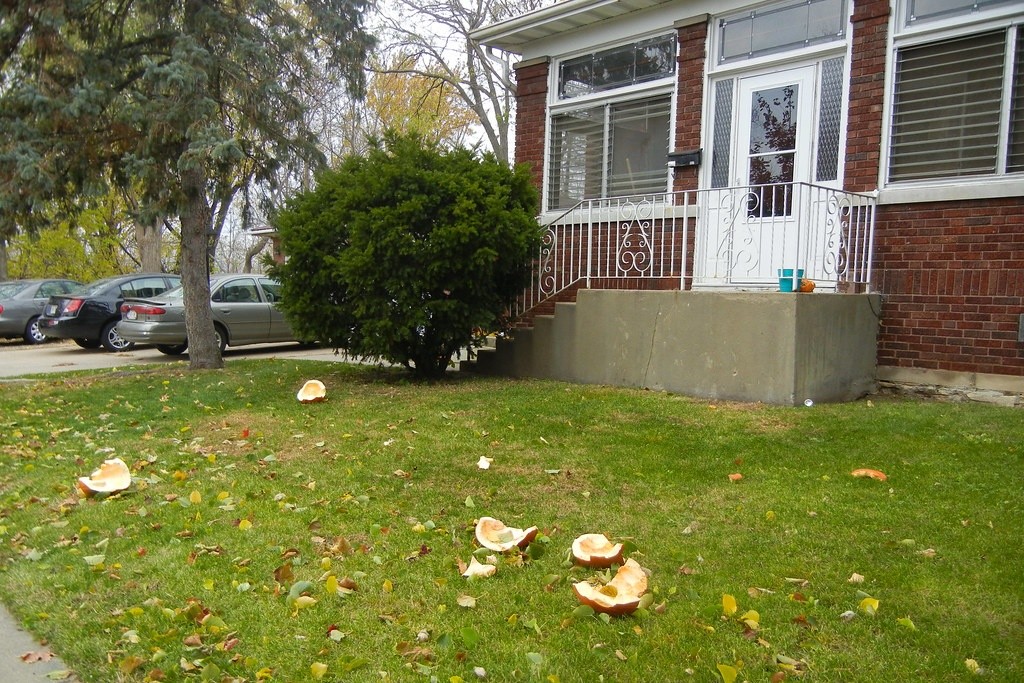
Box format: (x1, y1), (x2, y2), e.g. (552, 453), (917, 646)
(225, 285), (255, 303)
(143, 286), (154, 297)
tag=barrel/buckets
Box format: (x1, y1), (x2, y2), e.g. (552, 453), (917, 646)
(778, 269), (804, 292)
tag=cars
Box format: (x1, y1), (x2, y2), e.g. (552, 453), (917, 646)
(0, 279), (85, 343)
(38, 275), (181, 350)
(117, 274), (315, 357)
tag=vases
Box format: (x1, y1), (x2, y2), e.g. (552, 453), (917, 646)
(778, 269), (804, 292)
